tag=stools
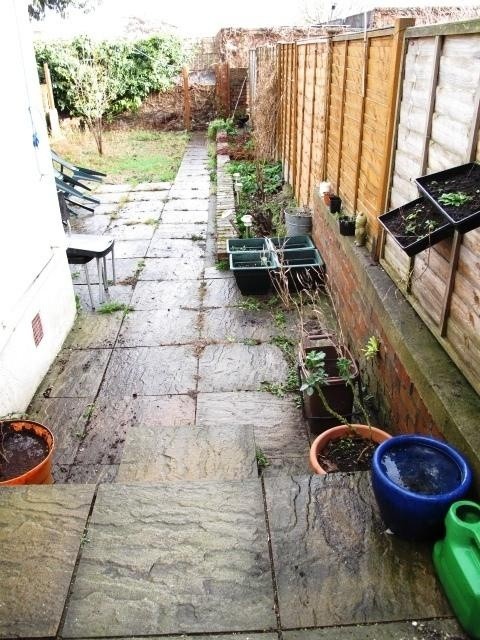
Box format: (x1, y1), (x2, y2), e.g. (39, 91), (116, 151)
(66, 233), (116, 290)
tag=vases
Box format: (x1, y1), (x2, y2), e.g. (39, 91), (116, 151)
(369, 432), (470, 541)
(225, 235), (325, 296)
(320, 181), (341, 213)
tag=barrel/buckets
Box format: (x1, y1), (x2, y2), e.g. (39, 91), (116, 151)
(284, 206), (312, 236)
(309, 424), (393, 474)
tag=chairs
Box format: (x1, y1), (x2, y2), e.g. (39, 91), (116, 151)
(55, 190), (104, 309)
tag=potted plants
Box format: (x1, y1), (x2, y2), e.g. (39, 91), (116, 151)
(334, 209), (356, 235)
(301, 348), (393, 475)
(376, 161), (480, 258)
